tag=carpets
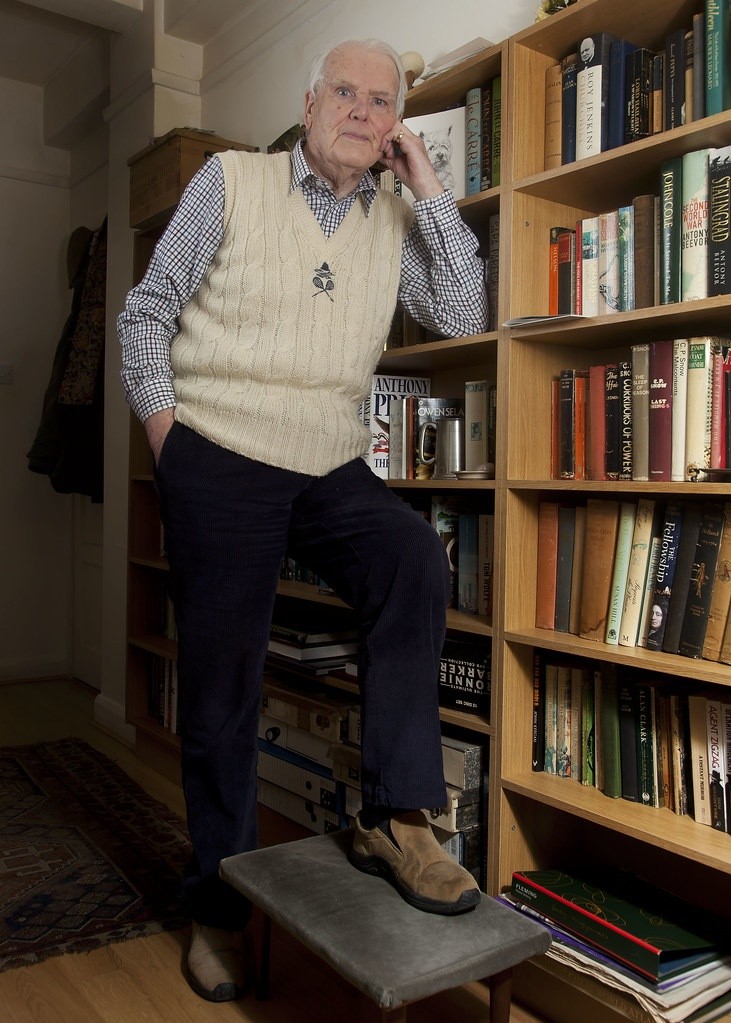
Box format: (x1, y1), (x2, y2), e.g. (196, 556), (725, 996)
(0, 738), (194, 970)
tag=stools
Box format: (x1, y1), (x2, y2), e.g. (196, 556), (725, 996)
(217, 830), (552, 1023)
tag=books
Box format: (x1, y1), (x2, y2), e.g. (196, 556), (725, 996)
(146, 0), (731, 833)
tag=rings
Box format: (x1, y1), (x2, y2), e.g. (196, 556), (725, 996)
(396, 131), (405, 142)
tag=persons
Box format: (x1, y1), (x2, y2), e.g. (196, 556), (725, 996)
(115, 38), (488, 1002)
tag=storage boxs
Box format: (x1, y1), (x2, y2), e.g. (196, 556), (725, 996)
(127, 127), (260, 229)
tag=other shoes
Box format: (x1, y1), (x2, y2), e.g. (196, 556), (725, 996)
(188, 922), (251, 1001)
(348, 803), (481, 915)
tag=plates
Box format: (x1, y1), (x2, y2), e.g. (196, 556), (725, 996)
(451, 469), (493, 479)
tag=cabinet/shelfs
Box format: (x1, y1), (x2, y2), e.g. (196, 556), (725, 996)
(124, 0), (731, 1023)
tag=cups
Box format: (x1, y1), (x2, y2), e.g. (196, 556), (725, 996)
(419, 416), (465, 480)
